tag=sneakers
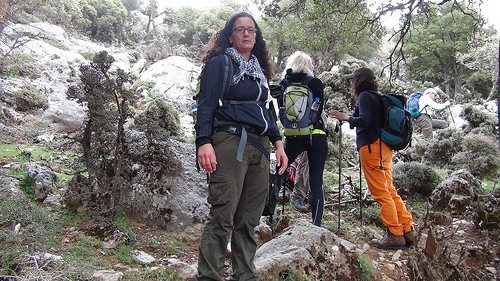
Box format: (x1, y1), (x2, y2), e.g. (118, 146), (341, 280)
(371, 226), (417, 250)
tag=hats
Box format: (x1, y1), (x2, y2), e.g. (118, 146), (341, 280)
(424, 88), (440, 100)
(343, 67), (377, 80)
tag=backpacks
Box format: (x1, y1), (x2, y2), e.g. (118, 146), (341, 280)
(406, 90), (429, 118)
(276, 67), (321, 135)
(357, 90), (414, 151)
(190, 50), (274, 162)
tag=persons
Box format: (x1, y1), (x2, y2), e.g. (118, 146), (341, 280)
(328, 67), (415, 250)
(192, 12), (289, 281)
(261, 51), (328, 227)
(407, 88), (451, 142)
(288, 108), (327, 214)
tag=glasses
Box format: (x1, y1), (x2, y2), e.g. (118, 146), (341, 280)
(232, 26), (259, 34)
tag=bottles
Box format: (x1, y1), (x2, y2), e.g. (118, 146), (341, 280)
(311, 97), (320, 111)
(191, 102), (197, 110)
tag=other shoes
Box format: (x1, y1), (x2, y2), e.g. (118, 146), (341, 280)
(290, 199), (308, 212)
(258, 215), (271, 226)
